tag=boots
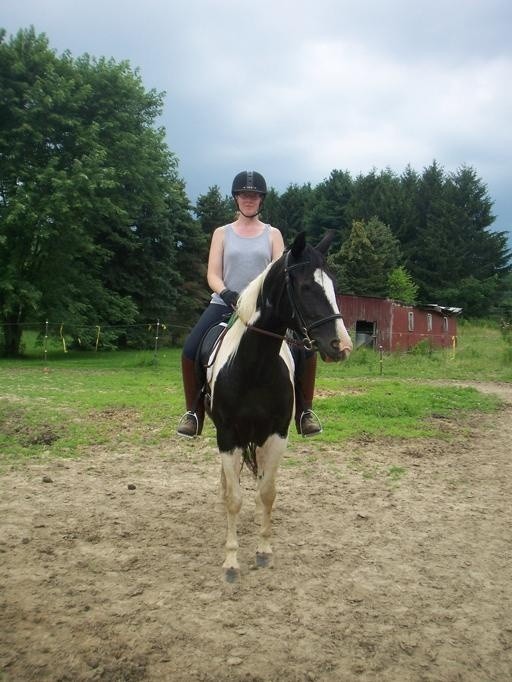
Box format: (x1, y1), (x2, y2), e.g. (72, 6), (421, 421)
(179, 356), (204, 436)
(296, 352), (319, 434)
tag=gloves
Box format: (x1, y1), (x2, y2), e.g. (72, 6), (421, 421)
(219, 288), (238, 306)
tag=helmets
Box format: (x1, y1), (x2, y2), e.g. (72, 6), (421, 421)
(232, 170), (268, 195)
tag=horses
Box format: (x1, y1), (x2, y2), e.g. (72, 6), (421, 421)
(194, 230), (353, 585)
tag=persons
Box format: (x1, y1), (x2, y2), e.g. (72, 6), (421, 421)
(177, 170), (321, 436)
